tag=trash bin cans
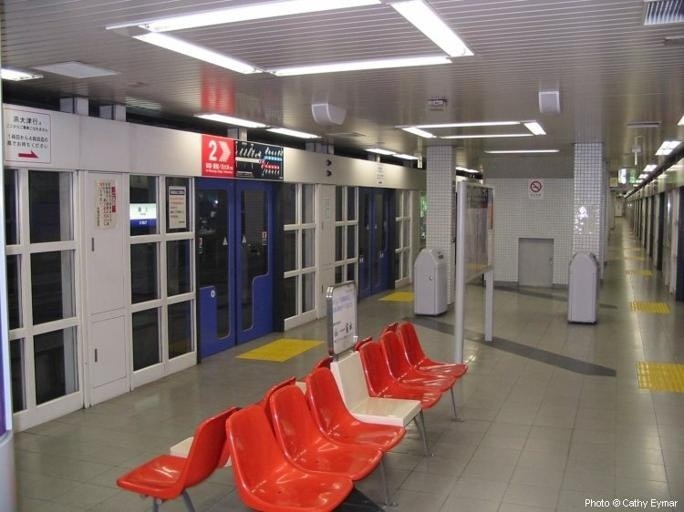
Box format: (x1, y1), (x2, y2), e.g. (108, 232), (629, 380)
(414, 248), (447, 317)
(568, 252), (599, 324)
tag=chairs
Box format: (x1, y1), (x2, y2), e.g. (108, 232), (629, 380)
(113, 322), (467, 512)
